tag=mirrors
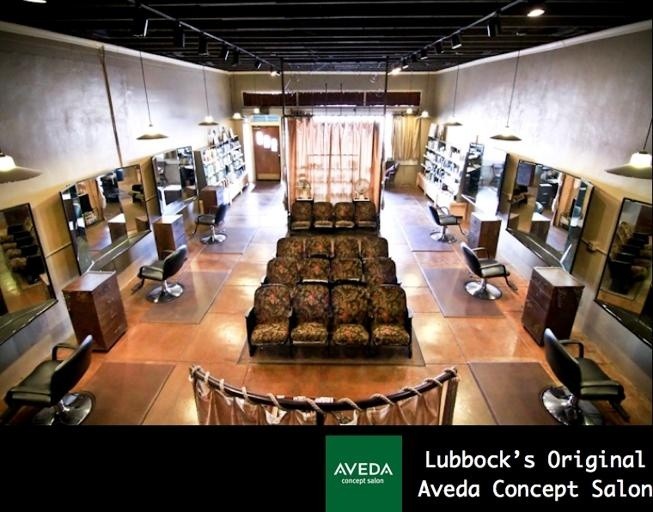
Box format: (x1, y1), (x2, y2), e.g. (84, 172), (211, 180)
(0, 146), (199, 345)
(253, 125), (280, 182)
(460, 143), (653, 350)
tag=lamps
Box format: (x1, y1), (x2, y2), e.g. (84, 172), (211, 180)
(489, 33), (527, 141)
(136, 39), (168, 139)
(199, 57), (219, 125)
(391, 0), (544, 73)
(605, 118), (653, 179)
(256, 130), (278, 153)
(406, 61), (431, 119)
(229, 67), (260, 120)
(131, 0), (281, 76)
(444, 52), (464, 126)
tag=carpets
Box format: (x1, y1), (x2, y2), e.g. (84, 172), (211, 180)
(141, 271), (230, 324)
(201, 227), (256, 254)
(468, 360), (557, 425)
(81, 362), (175, 425)
(422, 268), (504, 317)
(402, 225), (454, 252)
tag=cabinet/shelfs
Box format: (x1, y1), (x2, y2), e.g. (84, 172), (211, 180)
(194, 134), (249, 207)
(201, 186), (223, 214)
(62, 270), (128, 353)
(417, 135), (470, 221)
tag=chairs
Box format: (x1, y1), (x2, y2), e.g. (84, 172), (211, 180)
(244, 200), (413, 358)
(189, 204), (226, 244)
(0, 335), (93, 425)
(542, 327), (630, 425)
(427, 201), (466, 243)
